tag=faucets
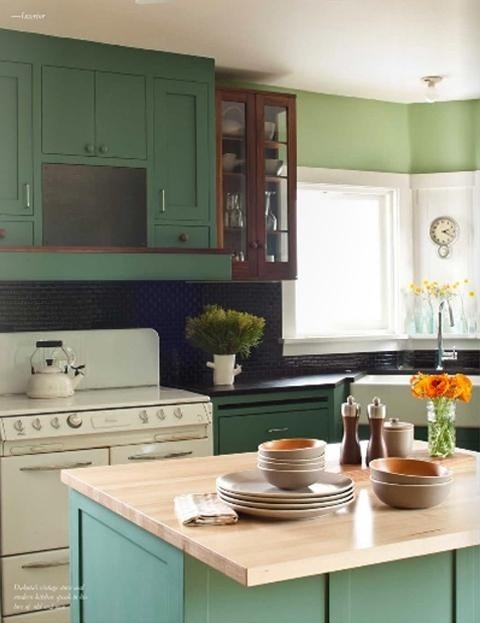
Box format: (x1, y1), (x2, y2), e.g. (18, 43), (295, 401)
(435, 299), (459, 371)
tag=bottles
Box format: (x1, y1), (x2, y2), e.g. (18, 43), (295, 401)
(366, 397), (386, 468)
(234, 251), (281, 263)
(341, 395), (362, 466)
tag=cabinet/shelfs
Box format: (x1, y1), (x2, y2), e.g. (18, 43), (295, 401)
(0, 29), (216, 248)
(215, 87), (296, 280)
(211, 384), (348, 455)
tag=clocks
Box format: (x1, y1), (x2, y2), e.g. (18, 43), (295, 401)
(429, 216), (460, 258)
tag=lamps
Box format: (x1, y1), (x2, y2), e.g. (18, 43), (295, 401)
(423, 76), (442, 103)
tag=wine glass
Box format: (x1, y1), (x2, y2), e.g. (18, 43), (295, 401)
(223, 190), (281, 229)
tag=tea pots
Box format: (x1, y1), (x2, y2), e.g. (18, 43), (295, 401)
(222, 104), (245, 138)
(26, 341), (85, 401)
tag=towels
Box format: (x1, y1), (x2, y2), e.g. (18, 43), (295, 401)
(173, 492), (238, 526)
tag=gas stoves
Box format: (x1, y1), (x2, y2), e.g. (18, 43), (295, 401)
(0, 385), (213, 440)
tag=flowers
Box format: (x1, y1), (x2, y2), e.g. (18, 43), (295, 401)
(410, 371), (473, 455)
(409, 279), (444, 312)
(444, 280), (476, 309)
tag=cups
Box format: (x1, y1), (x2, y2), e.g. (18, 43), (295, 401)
(381, 417), (415, 456)
(264, 122), (276, 140)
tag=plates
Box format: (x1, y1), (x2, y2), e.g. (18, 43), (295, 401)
(216, 468), (356, 520)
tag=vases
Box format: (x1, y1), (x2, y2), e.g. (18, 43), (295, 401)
(427, 304), (435, 335)
(461, 304), (469, 335)
(427, 400), (457, 457)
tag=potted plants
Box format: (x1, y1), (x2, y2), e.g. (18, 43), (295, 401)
(184, 303), (266, 388)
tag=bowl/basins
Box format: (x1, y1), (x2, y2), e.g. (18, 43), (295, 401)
(257, 439), (329, 489)
(367, 457), (453, 511)
(257, 158), (285, 174)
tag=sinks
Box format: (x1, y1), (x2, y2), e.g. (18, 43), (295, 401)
(350, 374), (480, 428)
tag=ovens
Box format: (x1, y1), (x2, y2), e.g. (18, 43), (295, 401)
(1, 434), (213, 611)
